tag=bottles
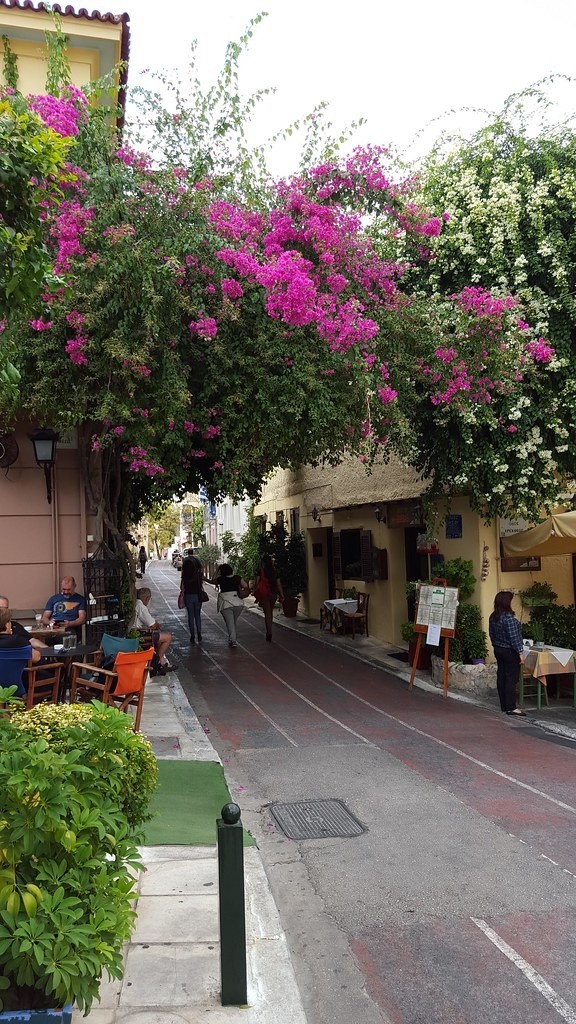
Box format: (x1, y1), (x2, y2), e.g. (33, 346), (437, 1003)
(35, 614), (43, 629)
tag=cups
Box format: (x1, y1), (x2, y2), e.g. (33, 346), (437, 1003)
(91, 614), (118, 622)
(537, 642), (544, 651)
(63, 635), (77, 651)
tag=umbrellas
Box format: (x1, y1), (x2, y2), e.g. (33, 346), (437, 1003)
(499, 511), (576, 558)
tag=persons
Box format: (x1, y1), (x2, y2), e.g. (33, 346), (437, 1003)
(180, 560), (203, 642)
(489, 591), (527, 716)
(130, 587), (171, 667)
(40, 575), (87, 647)
(0, 607), (41, 693)
(0, 596), (49, 648)
(138, 546), (147, 574)
(202, 563), (248, 647)
(181, 548), (201, 578)
(255, 554), (284, 642)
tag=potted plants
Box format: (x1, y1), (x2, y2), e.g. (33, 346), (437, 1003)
(241, 502), (307, 617)
(521, 581), (558, 607)
(400, 581), (432, 669)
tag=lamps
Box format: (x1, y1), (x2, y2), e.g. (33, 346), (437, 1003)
(374, 506), (386, 523)
(27, 419), (60, 504)
(278, 510), (288, 525)
(312, 507), (321, 523)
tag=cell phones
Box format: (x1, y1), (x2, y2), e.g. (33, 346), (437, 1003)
(54, 619), (64, 625)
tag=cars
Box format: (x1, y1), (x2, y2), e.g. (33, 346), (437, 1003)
(176, 547), (201, 571)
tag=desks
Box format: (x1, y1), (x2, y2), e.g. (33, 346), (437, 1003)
(325, 599), (358, 634)
(32, 645), (99, 702)
(29, 628), (66, 646)
(517, 643), (576, 710)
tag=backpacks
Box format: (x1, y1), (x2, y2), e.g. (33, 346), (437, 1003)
(88, 654), (117, 694)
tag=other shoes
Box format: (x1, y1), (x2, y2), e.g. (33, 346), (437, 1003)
(161, 663), (177, 672)
(229, 641), (238, 647)
(507, 709), (526, 716)
(190, 634), (202, 643)
(265, 631), (273, 640)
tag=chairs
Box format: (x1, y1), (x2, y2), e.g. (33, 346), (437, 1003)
(23, 624), (160, 734)
(319, 586), (370, 640)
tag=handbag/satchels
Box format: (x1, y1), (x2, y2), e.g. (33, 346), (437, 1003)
(200, 583), (209, 603)
(237, 576), (250, 598)
(256, 568), (271, 598)
(177, 592), (185, 610)
